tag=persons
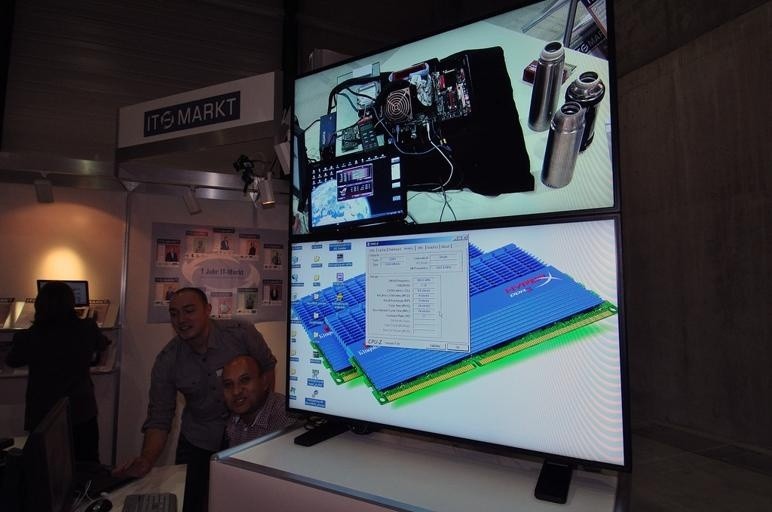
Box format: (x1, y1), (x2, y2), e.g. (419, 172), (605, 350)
(219, 353), (297, 451)
(272, 252), (281, 264)
(109, 286), (277, 512)
(6, 280), (106, 480)
(219, 301), (231, 313)
(165, 247), (177, 261)
(248, 242), (256, 255)
(221, 236), (229, 250)
(271, 286), (278, 300)
(166, 287), (174, 300)
(246, 295), (254, 308)
(196, 240), (205, 252)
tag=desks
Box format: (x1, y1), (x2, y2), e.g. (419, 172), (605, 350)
(204, 421), (625, 512)
(83, 463), (189, 512)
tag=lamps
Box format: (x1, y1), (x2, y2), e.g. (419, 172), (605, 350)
(254, 141), (290, 205)
(31, 173), (205, 216)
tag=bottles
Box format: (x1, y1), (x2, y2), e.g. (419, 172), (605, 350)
(526, 40), (606, 190)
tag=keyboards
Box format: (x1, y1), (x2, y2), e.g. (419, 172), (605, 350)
(122, 494), (176, 512)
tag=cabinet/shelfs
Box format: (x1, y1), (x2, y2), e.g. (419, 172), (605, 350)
(1, 325), (120, 378)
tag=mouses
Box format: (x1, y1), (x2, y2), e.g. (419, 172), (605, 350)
(85, 499), (113, 512)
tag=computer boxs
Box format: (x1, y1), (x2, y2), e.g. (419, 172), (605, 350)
(380, 47), (534, 195)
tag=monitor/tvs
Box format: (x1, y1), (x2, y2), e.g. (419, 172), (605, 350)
(288, 0), (622, 240)
(37, 280), (89, 307)
(285, 212), (633, 505)
(307, 145), (408, 233)
(22, 396), (74, 512)
